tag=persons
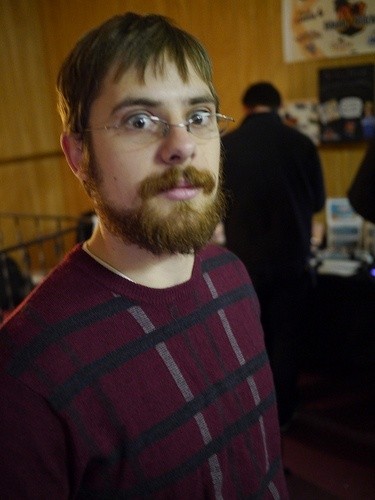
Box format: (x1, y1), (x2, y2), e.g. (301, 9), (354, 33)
(221, 81), (327, 432)
(0, 11), (289, 500)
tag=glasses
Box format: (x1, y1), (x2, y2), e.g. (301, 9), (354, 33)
(81, 113), (235, 145)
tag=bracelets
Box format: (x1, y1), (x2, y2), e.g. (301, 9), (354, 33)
(310, 239), (319, 253)
